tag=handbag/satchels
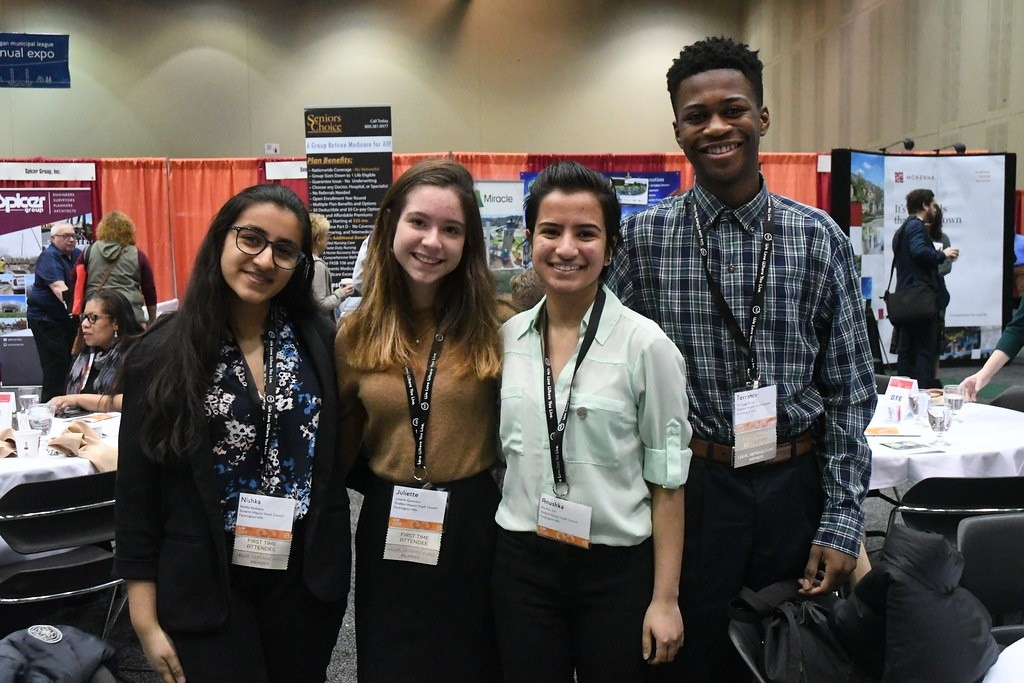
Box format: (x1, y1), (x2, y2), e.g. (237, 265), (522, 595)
(71, 327), (84, 357)
(884, 282), (937, 326)
(72, 244), (92, 315)
(726, 585), (866, 683)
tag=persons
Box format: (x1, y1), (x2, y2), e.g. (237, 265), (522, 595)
(957, 293), (1024, 401)
(893, 189), (959, 388)
(607, 35), (878, 683)
(116, 185), (352, 683)
(309, 213), (369, 324)
(26, 223), (82, 403)
(333, 160), (521, 683)
(74, 211), (157, 329)
(48, 289), (146, 416)
(494, 162), (692, 683)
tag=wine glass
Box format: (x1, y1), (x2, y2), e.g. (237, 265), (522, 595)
(926, 398), (953, 449)
(908, 388), (931, 427)
(17, 386), (39, 416)
(26, 403), (54, 459)
(943, 385), (965, 427)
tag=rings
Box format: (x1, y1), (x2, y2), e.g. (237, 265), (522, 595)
(831, 591), (838, 596)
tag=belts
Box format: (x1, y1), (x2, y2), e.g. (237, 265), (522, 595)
(687, 428), (818, 466)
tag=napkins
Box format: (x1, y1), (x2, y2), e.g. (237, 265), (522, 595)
(0, 428), (17, 459)
(48, 419), (118, 472)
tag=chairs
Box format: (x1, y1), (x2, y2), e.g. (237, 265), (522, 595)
(882, 476), (1024, 646)
(0, 471), (128, 683)
(989, 387), (1024, 412)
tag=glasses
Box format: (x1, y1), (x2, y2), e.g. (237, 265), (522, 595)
(79, 313), (113, 324)
(232, 225), (306, 270)
(54, 233), (78, 239)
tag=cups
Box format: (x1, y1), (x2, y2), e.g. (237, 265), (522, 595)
(339, 278), (353, 290)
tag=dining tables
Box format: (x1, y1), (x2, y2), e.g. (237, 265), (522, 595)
(0, 402), (120, 572)
(865, 394), (1024, 539)
